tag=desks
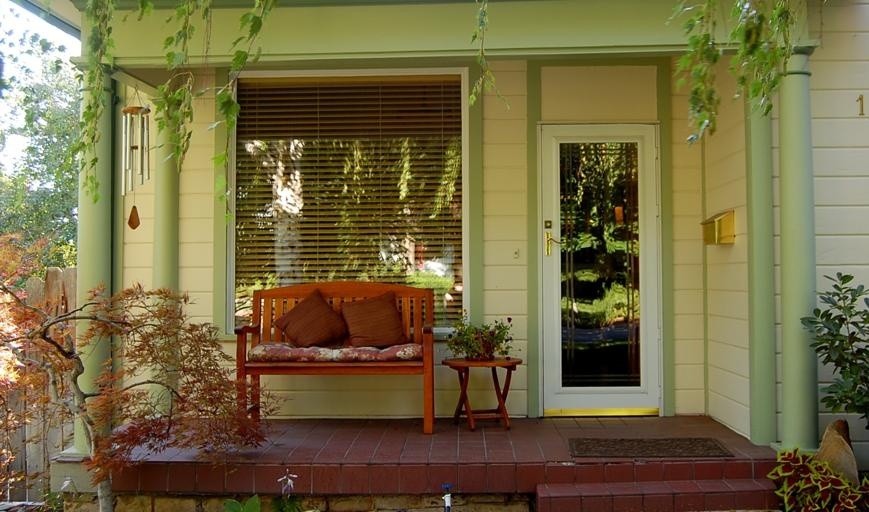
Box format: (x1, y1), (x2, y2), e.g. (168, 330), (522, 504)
(441, 357), (522, 431)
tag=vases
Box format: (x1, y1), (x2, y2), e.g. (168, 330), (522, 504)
(465, 352), (495, 360)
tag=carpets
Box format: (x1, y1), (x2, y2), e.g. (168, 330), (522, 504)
(567, 436), (736, 458)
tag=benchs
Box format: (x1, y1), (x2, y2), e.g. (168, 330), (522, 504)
(233, 281), (435, 433)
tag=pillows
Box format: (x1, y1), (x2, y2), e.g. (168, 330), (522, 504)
(272, 288), (348, 348)
(339, 290), (409, 347)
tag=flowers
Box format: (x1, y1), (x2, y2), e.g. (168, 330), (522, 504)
(446, 308), (512, 361)
